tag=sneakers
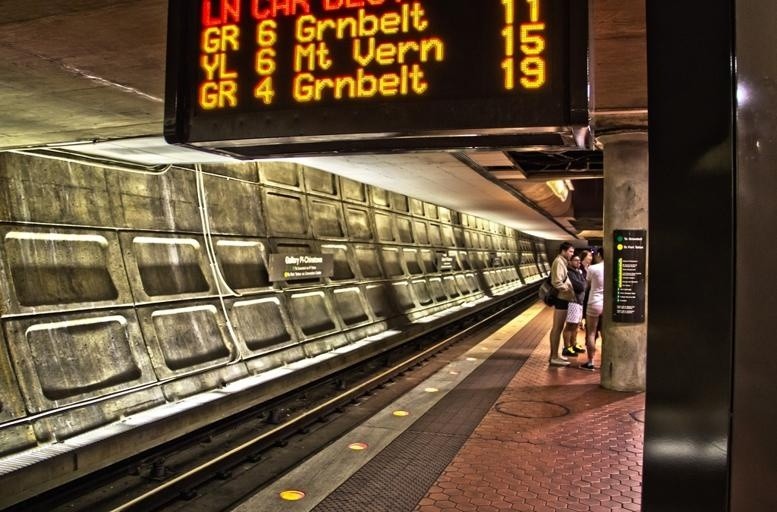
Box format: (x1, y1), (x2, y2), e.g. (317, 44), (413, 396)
(578, 362), (595, 371)
(550, 358), (571, 366)
(574, 343), (586, 353)
(562, 346), (579, 357)
(548, 355), (568, 361)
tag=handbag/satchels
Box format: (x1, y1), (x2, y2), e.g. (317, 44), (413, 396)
(539, 278), (560, 307)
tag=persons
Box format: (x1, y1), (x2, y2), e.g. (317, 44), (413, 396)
(550, 241), (604, 371)
